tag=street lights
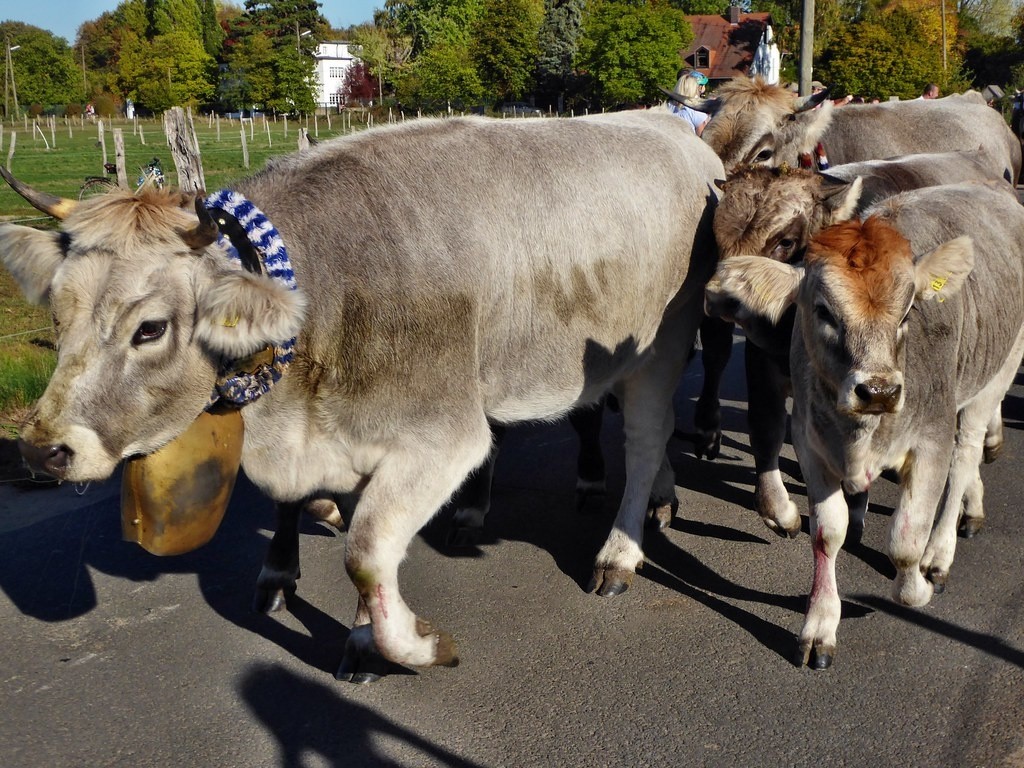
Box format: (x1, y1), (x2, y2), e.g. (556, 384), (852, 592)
(4, 45), (21, 119)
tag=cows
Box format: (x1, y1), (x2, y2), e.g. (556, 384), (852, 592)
(1, 107), (730, 688)
(643, 81), (1024, 462)
(702, 181), (1024, 672)
(702, 150), (1024, 539)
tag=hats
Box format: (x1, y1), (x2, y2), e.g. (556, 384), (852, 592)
(690, 71), (708, 85)
(811, 81), (827, 89)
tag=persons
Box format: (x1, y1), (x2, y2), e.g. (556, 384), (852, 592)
(661, 66), (717, 139)
(916, 83), (938, 100)
(783, 81), (881, 105)
(86, 103), (96, 120)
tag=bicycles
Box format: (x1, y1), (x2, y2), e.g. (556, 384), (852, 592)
(78, 156), (166, 204)
